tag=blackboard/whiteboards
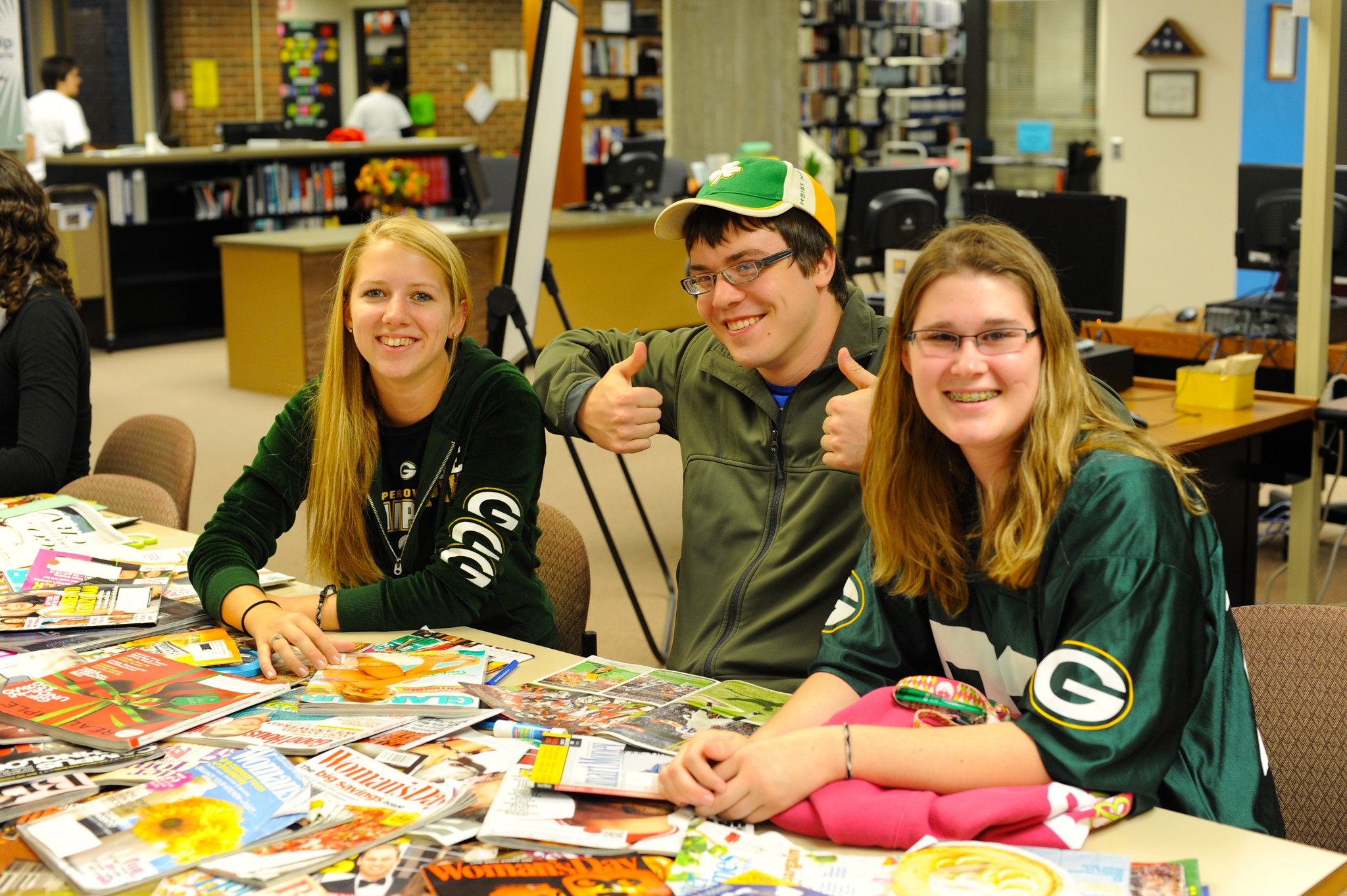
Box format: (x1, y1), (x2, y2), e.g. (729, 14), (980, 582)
(488, 0), (580, 371)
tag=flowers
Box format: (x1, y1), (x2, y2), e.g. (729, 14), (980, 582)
(356, 157), (430, 213)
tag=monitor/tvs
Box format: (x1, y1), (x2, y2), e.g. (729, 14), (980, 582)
(606, 136), (665, 206)
(961, 187), (1127, 350)
(1234, 164), (1347, 307)
(458, 144), (491, 225)
(841, 162), (951, 274)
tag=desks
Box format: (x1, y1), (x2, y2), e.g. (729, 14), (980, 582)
(1116, 376), (1321, 607)
(4, 492), (1347, 895)
(961, 186), (1128, 326)
(214, 209), (708, 398)
(1080, 308), (1347, 377)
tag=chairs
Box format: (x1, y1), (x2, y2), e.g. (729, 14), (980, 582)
(58, 155), (1347, 851)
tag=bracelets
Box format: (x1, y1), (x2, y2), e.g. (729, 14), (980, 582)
(316, 585), (337, 630)
(843, 722), (857, 780)
(241, 600), (282, 638)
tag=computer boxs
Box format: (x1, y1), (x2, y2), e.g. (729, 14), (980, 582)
(1203, 292), (1347, 344)
(1076, 341), (1133, 392)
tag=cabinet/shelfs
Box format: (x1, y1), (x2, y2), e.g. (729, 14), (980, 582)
(48, 135), (475, 350)
(661, 0), (968, 195)
(519, 0), (663, 206)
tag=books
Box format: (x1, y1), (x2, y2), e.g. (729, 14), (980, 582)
(0, 496), (1209, 896)
(107, 170), (147, 224)
(191, 159), (348, 232)
(366, 157), (452, 220)
(798, 0), (965, 188)
(580, 36), (661, 164)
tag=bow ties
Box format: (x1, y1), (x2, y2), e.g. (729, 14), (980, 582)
(359, 878), (386, 887)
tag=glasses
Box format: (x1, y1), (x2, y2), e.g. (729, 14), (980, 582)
(904, 328), (1039, 355)
(680, 247), (794, 295)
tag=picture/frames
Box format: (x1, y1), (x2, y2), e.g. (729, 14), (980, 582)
(1267, 3), (1299, 80)
(1145, 70), (1199, 118)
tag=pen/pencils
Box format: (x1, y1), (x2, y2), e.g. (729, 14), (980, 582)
(484, 659), (519, 686)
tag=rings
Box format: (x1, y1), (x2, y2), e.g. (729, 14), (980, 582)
(271, 634), (286, 649)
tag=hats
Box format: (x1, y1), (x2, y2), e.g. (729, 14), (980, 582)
(654, 157), (836, 248)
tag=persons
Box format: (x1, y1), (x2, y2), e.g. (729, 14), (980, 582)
(188, 219), (558, 680)
(349, 65), (414, 141)
(24, 55), (96, 187)
(533, 156), (892, 694)
(0, 150), (92, 499)
(659, 221), (1287, 838)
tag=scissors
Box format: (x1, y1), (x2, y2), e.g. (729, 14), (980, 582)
(123, 532), (157, 548)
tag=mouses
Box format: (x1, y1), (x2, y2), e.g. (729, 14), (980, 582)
(1131, 412), (1148, 427)
(1176, 308), (1198, 322)
